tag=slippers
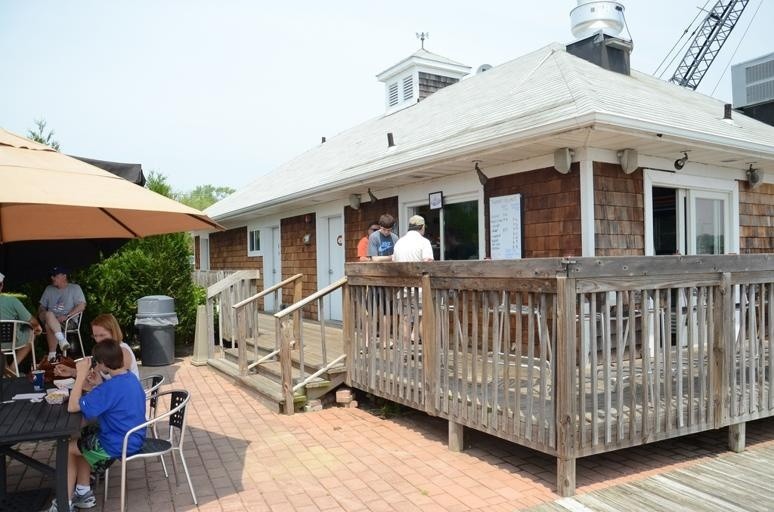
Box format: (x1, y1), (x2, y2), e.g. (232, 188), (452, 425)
(360, 338), (422, 355)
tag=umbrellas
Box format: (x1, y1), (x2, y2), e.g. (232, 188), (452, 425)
(0, 124), (228, 252)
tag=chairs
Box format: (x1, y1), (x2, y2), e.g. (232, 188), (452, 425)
(89, 386), (199, 512)
(132, 374), (175, 477)
(1, 315), (41, 375)
(60, 311), (84, 361)
(426, 288), (770, 383)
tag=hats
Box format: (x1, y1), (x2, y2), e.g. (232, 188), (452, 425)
(47, 265), (69, 275)
(0, 272), (5, 282)
(408, 214), (426, 226)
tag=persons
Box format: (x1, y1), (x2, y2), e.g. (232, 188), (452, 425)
(45, 339), (147, 511)
(357, 213), (434, 364)
(53, 314), (140, 390)
(0, 272), (42, 378)
(38, 265), (87, 363)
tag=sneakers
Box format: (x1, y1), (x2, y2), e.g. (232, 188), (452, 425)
(58, 338), (71, 351)
(42, 498), (74, 512)
(72, 489), (97, 509)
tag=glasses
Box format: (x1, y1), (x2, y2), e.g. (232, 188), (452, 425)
(50, 273), (60, 277)
(370, 228), (379, 232)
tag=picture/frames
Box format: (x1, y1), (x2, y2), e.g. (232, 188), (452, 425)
(427, 192), (445, 211)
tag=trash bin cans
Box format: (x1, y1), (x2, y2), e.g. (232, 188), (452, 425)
(134, 295), (179, 366)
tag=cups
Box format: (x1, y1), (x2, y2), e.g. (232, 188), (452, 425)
(31, 371), (46, 391)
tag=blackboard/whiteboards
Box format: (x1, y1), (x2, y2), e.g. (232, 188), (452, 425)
(488, 194), (524, 259)
